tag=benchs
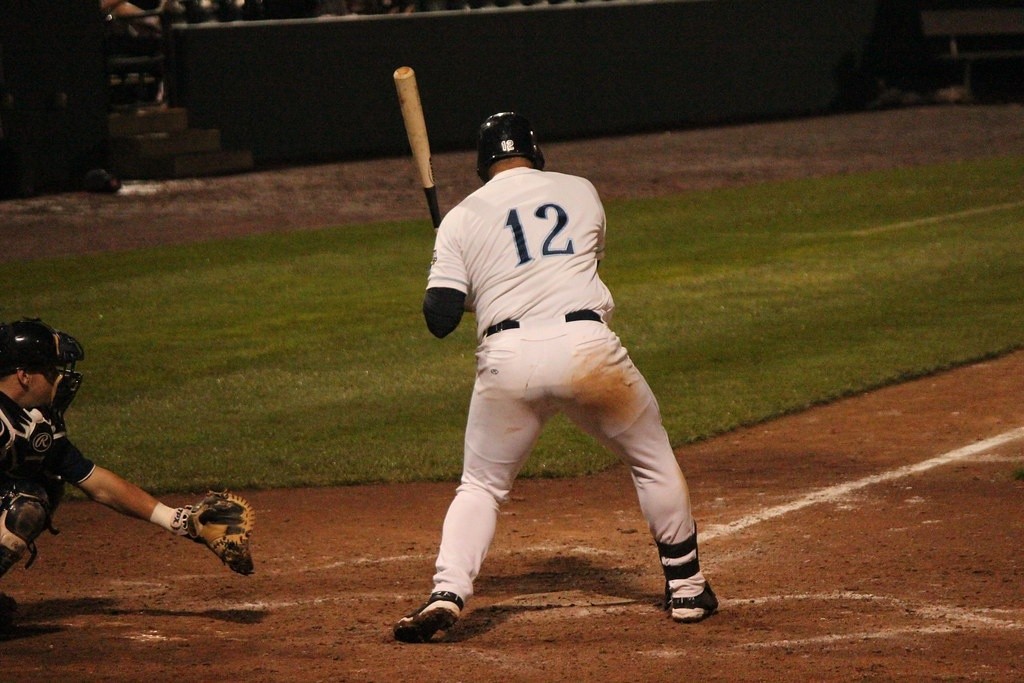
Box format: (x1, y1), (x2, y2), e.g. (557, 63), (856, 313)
(920, 7), (1024, 103)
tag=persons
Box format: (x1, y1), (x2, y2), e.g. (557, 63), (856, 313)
(0, 316), (245, 613)
(392, 111), (719, 644)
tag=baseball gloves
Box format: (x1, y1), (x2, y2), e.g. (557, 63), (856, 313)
(177, 488), (257, 576)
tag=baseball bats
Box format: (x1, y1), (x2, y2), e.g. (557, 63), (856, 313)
(393, 65), (443, 233)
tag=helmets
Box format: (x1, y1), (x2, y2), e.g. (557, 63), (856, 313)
(475, 112), (547, 172)
(0, 316), (84, 415)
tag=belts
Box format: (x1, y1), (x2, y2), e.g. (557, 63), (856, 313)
(486, 308), (603, 337)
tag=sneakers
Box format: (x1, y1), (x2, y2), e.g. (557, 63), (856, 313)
(669, 583), (720, 623)
(395, 591), (463, 641)
